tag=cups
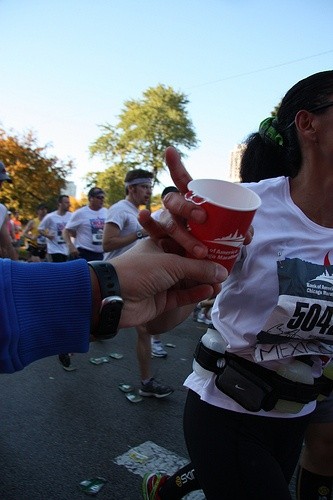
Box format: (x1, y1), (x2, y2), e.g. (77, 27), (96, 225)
(185, 179), (262, 276)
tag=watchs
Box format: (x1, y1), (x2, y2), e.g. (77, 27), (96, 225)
(136, 230), (143, 239)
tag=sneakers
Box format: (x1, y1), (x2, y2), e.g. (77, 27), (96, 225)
(151, 339), (168, 357)
(193, 306), (200, 321)
(139, 377), (173, 397)
(198, 313), (213, 324)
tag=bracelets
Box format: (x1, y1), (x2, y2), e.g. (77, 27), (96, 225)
(87, 261), (124, 340)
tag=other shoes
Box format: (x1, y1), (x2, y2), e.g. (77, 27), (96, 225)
(141, 470), (170, 500)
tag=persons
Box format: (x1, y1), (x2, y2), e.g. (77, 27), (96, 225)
(101, 169), (175, 398)
(150, 186), (179, 222)
(7, 209), (22, 246)
(139, 70), (333, 500)
(0, 236), (228, 374)
(192, 296), (212, 325)
(0, 160), (18, 261)
(21, 187), (108, 263)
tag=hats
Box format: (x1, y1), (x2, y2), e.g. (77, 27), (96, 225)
(0, 162), (13, 183)
